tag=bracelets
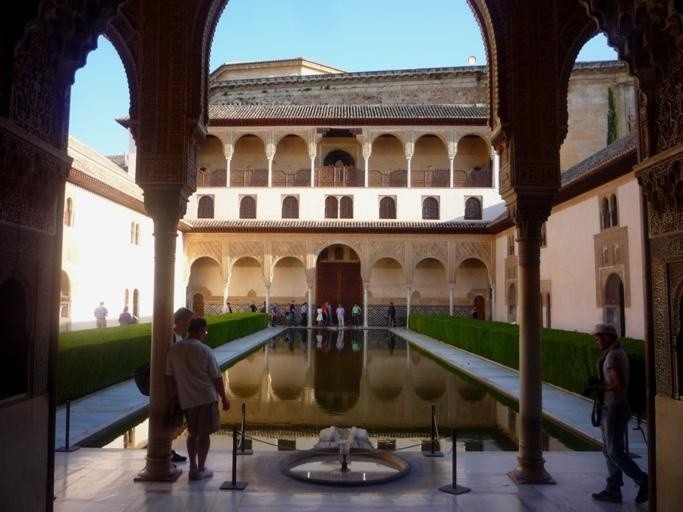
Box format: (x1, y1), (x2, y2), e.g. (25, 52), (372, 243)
(169, 398), (178, 401)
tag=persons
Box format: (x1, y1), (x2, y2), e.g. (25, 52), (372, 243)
(471, 305), (478, 319)
(165, 316), (230, 482)
(117, 305), (131, 326)
(585, 322), (648, 505)
(170, 308), (194, 469)
(93, 301), (109, 328)
(225, 300), (397, 328)
(262, 329), (396, 356)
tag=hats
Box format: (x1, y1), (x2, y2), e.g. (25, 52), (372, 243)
(590, 324), (618, 339)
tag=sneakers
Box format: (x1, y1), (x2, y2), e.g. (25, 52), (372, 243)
(634, 471), (651, 504)
(170, 449), (187, 463)
(188, 466), (214, 479)
(592, 490), (624, 503)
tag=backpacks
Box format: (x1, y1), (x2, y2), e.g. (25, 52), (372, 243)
(134, 359), (151, 396)
(627, 352), (648, 419)
